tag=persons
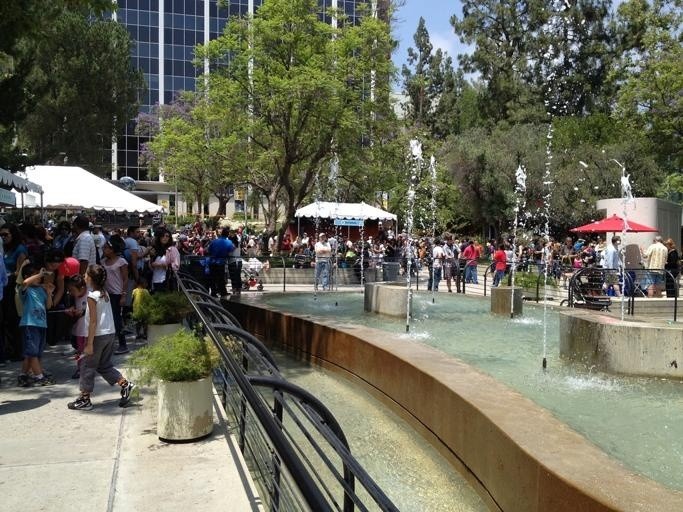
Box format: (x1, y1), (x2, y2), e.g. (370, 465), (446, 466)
(0, 206), (682, 408)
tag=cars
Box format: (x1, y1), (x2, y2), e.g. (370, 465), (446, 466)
(231, 209), (250, 219)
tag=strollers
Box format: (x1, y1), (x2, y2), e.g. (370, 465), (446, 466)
(560, 265), (613, 312)
(234, 258), (270, 291)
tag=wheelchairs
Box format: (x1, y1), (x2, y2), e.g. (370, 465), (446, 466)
(452, 257), (469, 282)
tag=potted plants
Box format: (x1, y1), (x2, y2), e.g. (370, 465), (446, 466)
(124, 329), (244, 443)
(130, 285), (204, 349)
(498, 269), (559, 302)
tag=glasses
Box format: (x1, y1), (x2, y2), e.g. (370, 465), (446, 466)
(0, 233), (11, 236)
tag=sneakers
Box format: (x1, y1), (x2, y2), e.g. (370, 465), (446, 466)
(68, 400), (93, 411)
(114, 348), (129, 354)
(72, 370), (80, 378)
(135, 336), (147, 346)
(119, 382), (136, 407)
(18, 369), (56, 387)
